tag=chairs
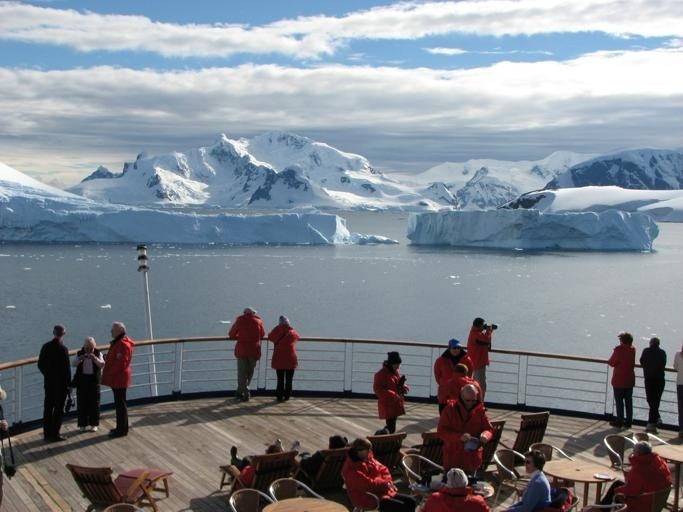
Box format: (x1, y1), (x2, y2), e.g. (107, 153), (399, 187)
(97, 501), (143, 512)
(64, 462), (184, 512)
(219, 412), (682, 511)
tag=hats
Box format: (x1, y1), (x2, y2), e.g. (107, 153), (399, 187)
(388, 351), (401, 364)
(464, 437), (482, 452)
(447, 468), (469, 488)
(449, 339), (461, 348)
(280, 316), (290, 325)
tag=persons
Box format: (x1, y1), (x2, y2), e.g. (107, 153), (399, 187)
(639, 336), (667, 435)
(672, 344), (683, 439)
(99, 321), (136, 439)
(71, 336), (106, 433)
(0, 385), (9, 509)
(598, 440), (673, 512)
(35, 324), (74, 444)
(607, 331), (637, 428)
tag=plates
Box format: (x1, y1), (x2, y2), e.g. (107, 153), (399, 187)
(593, 472), (612, 480)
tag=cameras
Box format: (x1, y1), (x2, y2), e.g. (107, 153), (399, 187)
(485, 324), (497, 329)
(4, 466), (16, 480)
